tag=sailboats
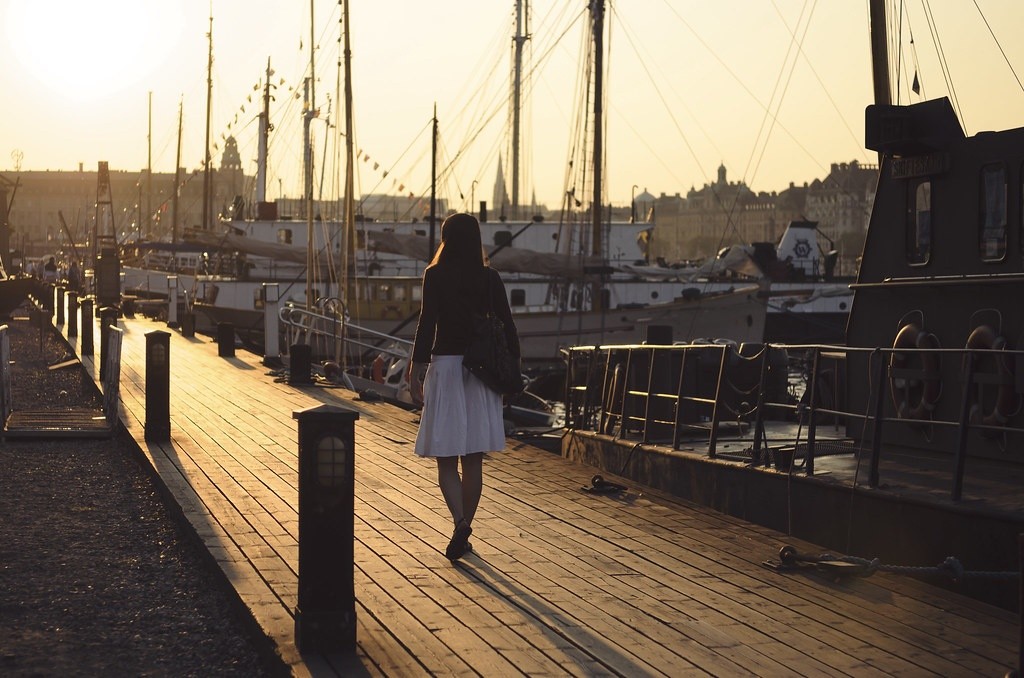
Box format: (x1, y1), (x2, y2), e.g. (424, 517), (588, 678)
(0, 1), (861, 404)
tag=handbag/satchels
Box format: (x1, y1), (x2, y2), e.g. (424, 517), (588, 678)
(461, 267), (522, 394)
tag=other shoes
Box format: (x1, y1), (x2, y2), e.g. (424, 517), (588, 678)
(446, 519), (473, 559)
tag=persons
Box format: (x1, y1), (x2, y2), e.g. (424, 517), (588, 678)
(409, 213), (520, 561)
(27, 260), (33, 273)
(68, 263), (80, 291)
(45, 257), (58, 282)
(37, 259), (44, 279)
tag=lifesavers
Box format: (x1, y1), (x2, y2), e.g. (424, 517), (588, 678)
(382, 304), (403, 319)
(888, 323), (941, 430)
(373, 347), (410, 389)
(962, 325), (1016, 439)
